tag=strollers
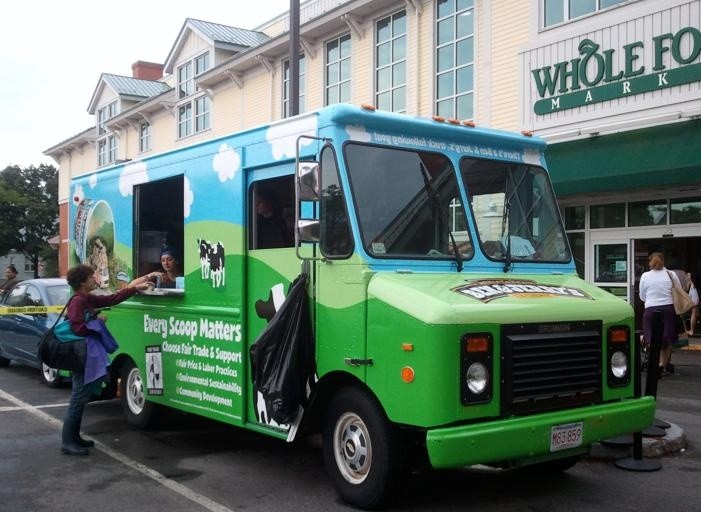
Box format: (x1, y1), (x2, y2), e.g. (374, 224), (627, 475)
(640, 334), (675, 374)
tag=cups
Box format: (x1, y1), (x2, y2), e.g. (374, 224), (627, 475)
(175, 277), (184, 289)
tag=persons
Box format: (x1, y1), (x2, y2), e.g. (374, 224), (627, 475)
(0, 266), (20, 294)
(258, 194), (293, 249)
(638, 251), (701, 377)
(60, 265), (155, 456)
(126, 247), (183, 288)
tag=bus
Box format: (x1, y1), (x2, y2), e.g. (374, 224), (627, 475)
(67, 106), (656, 505)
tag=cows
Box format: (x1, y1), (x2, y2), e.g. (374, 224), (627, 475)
(206, 241), (225, 288)
(197, 238), (212, 279)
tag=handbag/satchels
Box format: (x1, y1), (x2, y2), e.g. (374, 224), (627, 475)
(672, 287), (696, 315)
(37, 328), (87, 370)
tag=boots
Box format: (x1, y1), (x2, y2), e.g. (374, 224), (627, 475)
(61, 416), (95, 455)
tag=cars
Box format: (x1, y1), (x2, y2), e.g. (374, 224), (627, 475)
(0, 279), (77, 387)
